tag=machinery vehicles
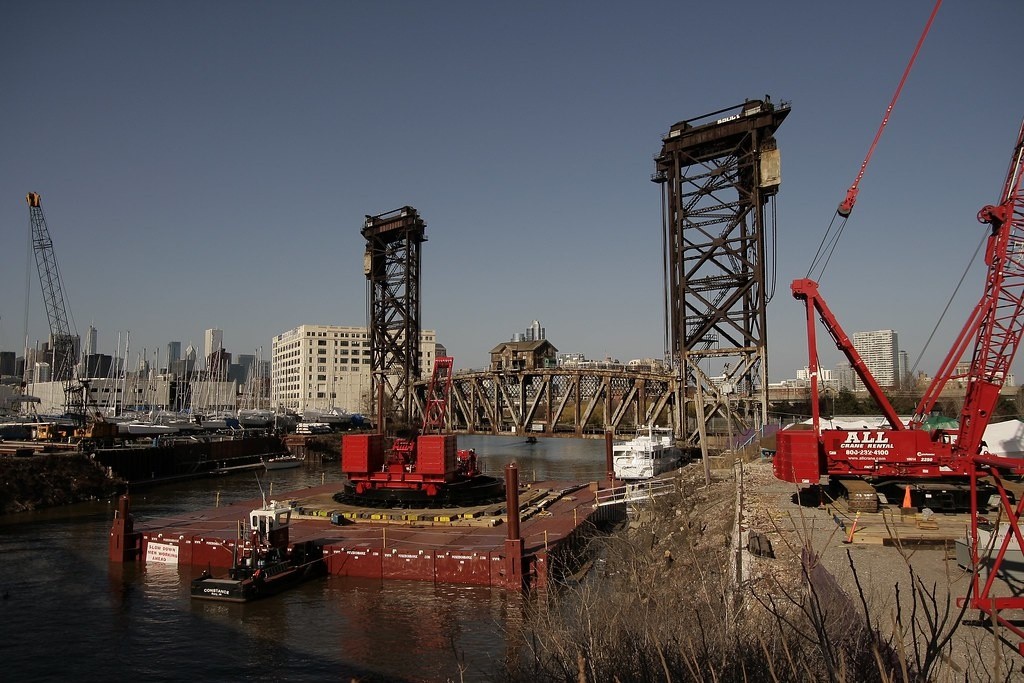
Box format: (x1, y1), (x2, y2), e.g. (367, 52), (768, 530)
(773, 0), (1024, 513)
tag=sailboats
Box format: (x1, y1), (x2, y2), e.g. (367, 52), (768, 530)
(118, 318), (275, 435)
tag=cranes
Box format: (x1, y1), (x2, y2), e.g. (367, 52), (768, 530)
(24, 191), (117, 450)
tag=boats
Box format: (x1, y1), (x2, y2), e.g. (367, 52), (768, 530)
(612, 410), (682, 479)
